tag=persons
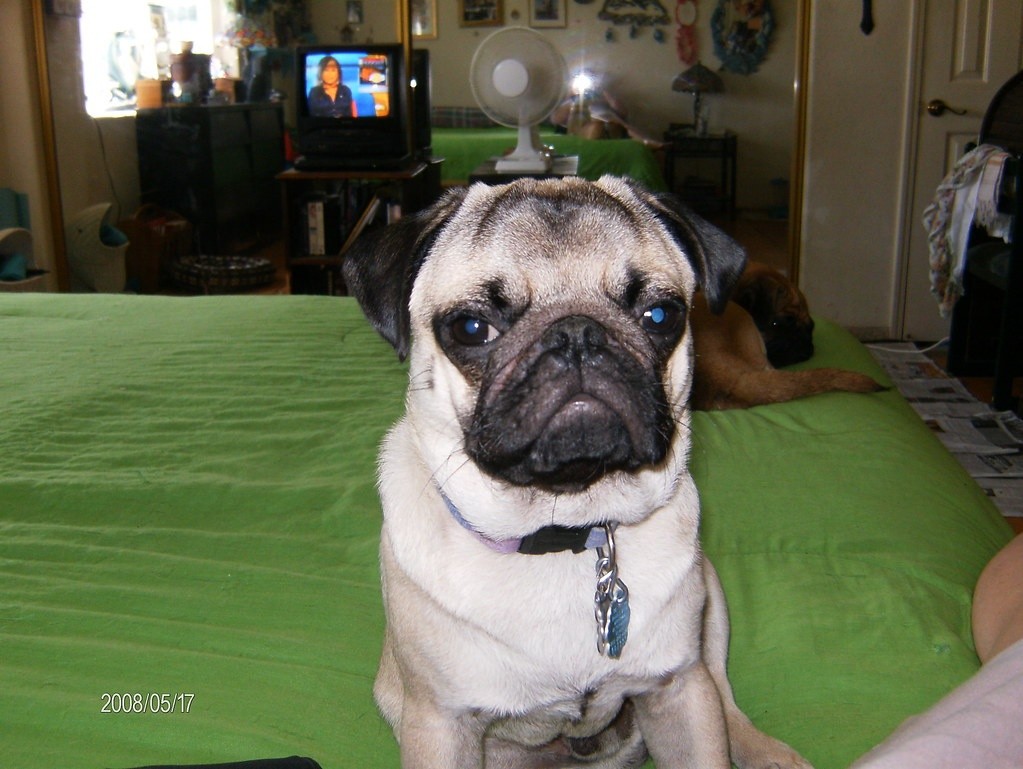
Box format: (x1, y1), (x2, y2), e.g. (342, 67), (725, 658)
(307, 56), (352, 117)
(551, 67), (663, 148)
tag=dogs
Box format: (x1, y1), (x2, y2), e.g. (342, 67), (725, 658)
(341, 170), (897, 769)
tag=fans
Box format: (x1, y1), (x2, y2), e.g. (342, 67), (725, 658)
(471, 25), (568, 170)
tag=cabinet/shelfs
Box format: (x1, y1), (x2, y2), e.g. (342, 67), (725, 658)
(426, 153), (445, 205)
(136, 100), (284, 255)
(275, 157), (428, 295)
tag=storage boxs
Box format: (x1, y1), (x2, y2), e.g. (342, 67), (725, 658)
(113, 203), (190, 293)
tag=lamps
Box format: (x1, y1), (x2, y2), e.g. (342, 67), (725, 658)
(672, 59), (726, 129)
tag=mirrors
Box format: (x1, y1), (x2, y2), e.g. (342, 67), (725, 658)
(33, 1), (402, 294)
(401, 0), (811, 297)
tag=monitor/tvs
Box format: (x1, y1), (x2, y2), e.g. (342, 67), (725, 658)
(293, 46), (410, 157)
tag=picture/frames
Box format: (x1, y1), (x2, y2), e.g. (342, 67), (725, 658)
(459, 0), (505, 29)
(411, 1), (436, 41)
(529, 0), (568, 29)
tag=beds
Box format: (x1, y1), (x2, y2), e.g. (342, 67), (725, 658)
(0, 293), (1023, 768)
(432, 122), (669, 203)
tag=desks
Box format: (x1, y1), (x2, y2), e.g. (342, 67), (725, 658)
(942, 156), (1022, 415)
(468, 155), (567, 185)
(662, 126), (737, 218)
